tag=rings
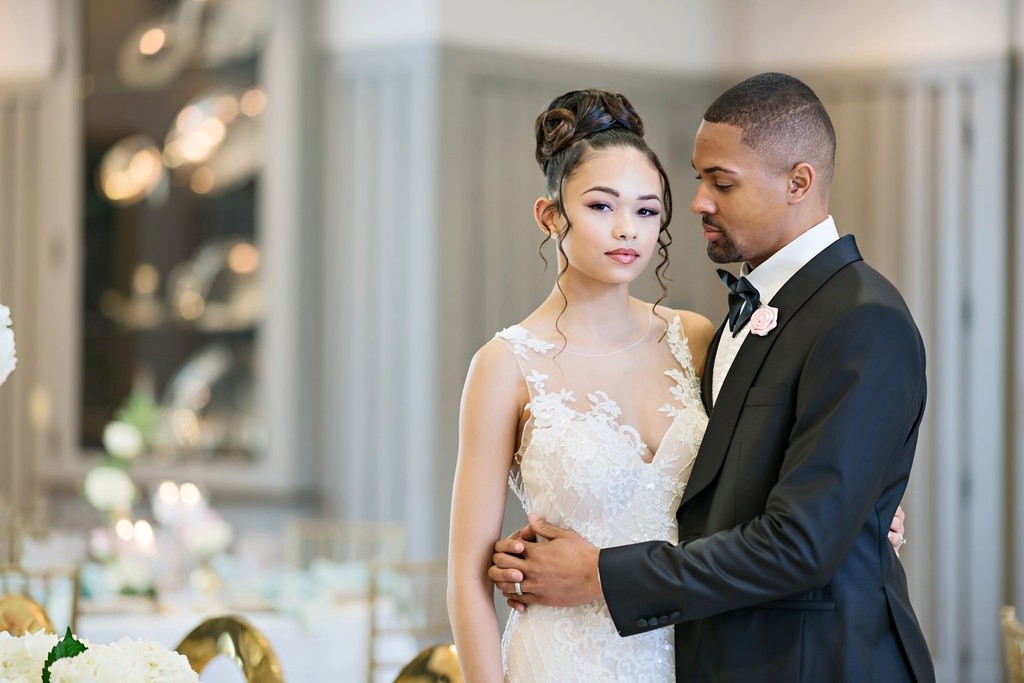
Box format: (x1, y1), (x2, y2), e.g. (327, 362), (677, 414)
(897, 535), (906, 547)
(515, 583), (523, 596)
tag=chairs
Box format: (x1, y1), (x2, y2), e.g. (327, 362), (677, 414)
(368, 538), (448, 683)
(0, 562), (81, 637)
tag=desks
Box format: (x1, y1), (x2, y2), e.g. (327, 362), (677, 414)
(31, 558), (425, 683)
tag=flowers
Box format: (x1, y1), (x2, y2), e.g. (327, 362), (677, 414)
(0, 627), (199, 683)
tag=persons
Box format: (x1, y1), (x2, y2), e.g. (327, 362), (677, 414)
(446, 89), (906, 683)
(489, 72), (935, 683)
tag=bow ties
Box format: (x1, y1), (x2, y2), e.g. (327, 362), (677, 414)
(715, 268), (760, 339)
